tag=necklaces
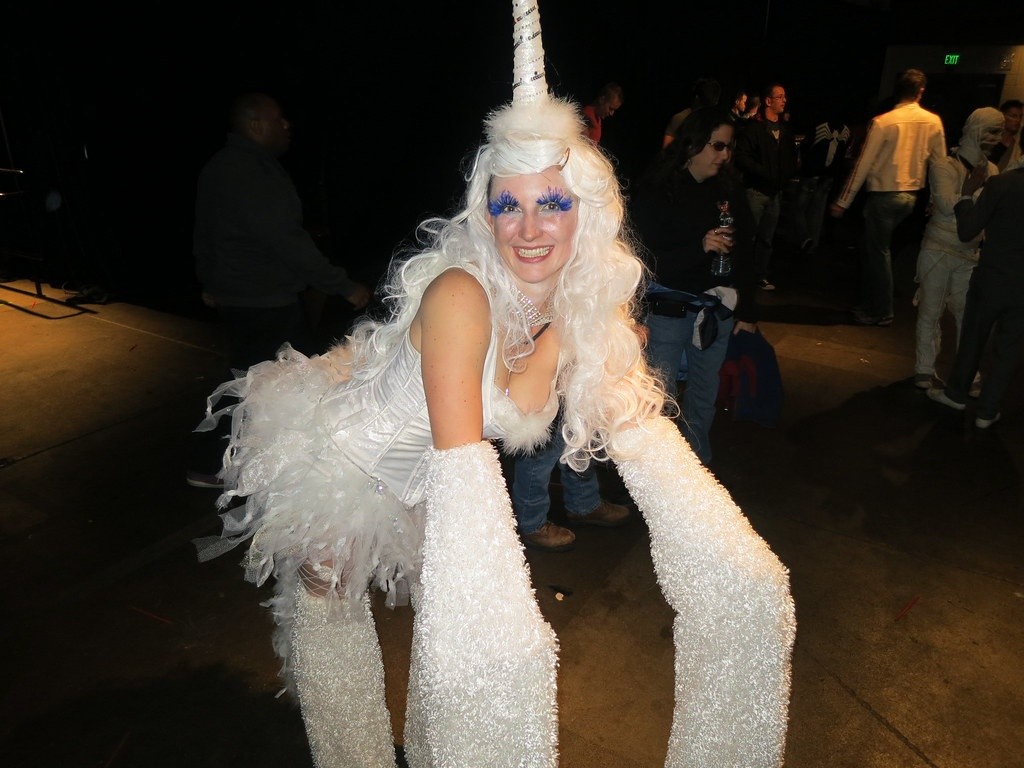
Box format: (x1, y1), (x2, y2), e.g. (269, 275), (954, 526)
(505, 286), (559, 396)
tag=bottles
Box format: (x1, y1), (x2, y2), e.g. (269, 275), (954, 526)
(710, 201), (734, 275)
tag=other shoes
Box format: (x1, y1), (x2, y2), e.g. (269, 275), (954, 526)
(852, 310), (894, 325)
(915, 372), (947, 389)
(755, 279), (775, 290)
(967, 374), (982, 398)
(926, 388), (966, 410)
(807, 246), (817, 254)
(975, 412), (1001, 429)
(186, 473), (224, 489)
(800, 237), (813, 250)
(566, 500), (631, 527)
(523, 520), (577, 552)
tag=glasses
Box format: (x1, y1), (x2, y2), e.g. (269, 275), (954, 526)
(706, 141), (734, 152)
(770, 95), (787, 101)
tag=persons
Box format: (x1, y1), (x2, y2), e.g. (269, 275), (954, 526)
(184, 96), (370, 489)
(511, 68), (1024, 551)
(193, 93), (798, 768)
(580, 82), (624, 144)
